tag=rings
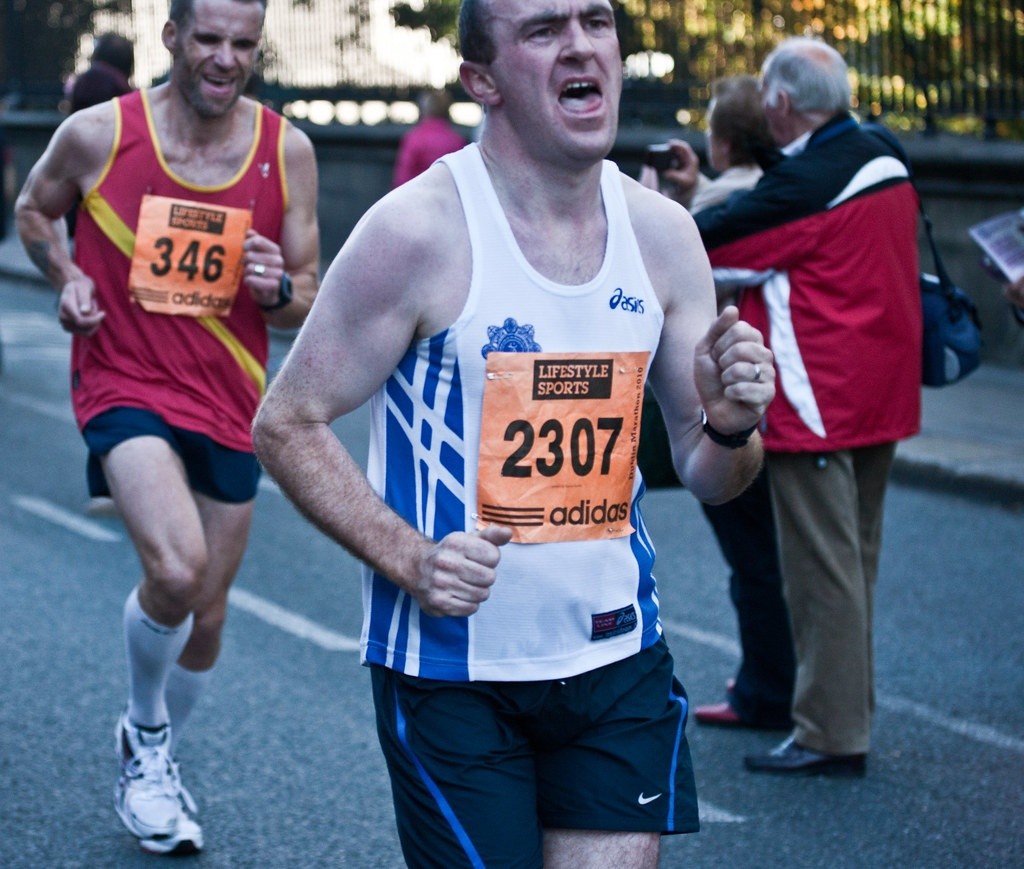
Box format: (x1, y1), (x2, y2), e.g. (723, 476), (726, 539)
(755, 364), (760, 380)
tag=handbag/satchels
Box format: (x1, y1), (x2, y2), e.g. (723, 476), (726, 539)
(861, 127), (983, 388)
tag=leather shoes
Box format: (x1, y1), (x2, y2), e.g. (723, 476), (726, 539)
(743, 735), (867, 777)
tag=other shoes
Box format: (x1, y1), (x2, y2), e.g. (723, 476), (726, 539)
(694, 703), (739, 723)
(727, 679), (734, 690)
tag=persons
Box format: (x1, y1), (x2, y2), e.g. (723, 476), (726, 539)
(395, 91), (472, 187)
(14, 0), (320, 855)
(693, 39), (923, 779)
(251, 0), (776, 869)
(62, 31), (134, 238)
(660, 75), (796, 729)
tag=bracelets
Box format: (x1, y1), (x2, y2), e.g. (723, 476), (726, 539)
(700, 408), (760, 448)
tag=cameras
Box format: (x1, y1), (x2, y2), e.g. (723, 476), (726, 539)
(646, 144), (674, 172)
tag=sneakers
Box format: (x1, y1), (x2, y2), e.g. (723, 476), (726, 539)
(113, 700), (198, 839)
(135, 762), (203, 857)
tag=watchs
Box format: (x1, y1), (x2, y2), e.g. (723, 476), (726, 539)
(255, 273), (294, 311)
